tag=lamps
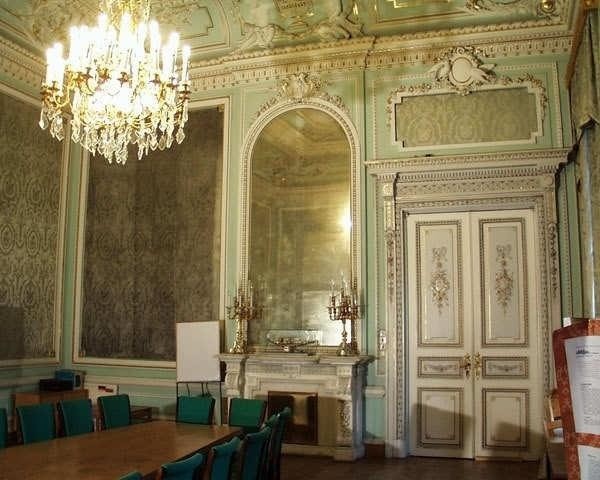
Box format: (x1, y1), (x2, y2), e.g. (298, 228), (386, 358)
(328, 270), (362, 358)
(38, 0), (191, 165)
(225, 273), (258, 354)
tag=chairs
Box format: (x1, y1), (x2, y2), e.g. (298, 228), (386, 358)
(0, 394), (292, 480)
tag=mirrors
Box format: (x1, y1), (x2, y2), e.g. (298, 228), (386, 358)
(238, 72), (361, 357)
